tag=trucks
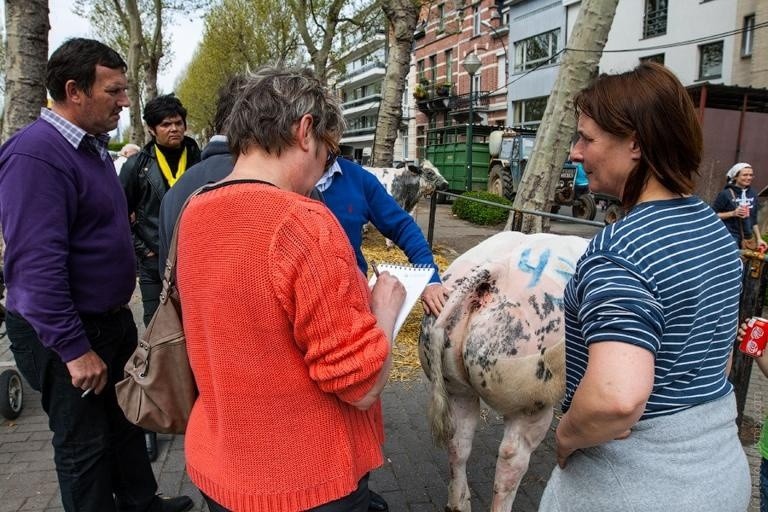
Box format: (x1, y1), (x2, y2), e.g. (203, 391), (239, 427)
(424, 123), (597, 220)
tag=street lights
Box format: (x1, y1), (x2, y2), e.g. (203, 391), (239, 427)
(481, 20), (509, 88)
(462, 54), (482, 192)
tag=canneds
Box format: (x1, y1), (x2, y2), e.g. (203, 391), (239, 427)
(738, 316), (768, 359)
(757, 245), (764, 254)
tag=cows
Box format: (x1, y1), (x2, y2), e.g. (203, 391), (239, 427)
(417, 230), (594, 512)
(362, 158), (449, 252)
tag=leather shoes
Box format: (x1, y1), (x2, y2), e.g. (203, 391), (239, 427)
(144, 432), (158, 463)
(151, 493), (194, 512)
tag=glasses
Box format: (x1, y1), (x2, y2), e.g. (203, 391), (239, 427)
(325, 143), (337, 172)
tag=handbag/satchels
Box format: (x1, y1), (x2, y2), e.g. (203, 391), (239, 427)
(741, 234), (758, 250)
(114, 282), (196, 435)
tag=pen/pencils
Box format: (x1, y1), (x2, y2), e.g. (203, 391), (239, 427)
(370, 260), (379, 277)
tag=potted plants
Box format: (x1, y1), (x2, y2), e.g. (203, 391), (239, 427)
(412, 76), (454, 101)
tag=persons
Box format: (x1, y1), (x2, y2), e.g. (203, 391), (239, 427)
(573, 163), (590, 198)
(0, 38), (193, 512)
(737, 318), (768, 512)
(538, 64), (752, 512)
(713, 163), (768, 271)
(109, 65), (450, 512)
